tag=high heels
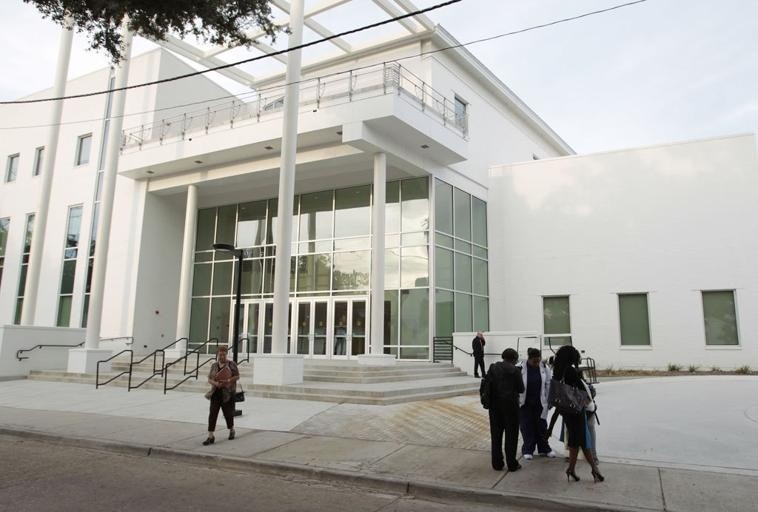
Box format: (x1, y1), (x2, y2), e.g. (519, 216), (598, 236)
(592, 468), (603, 482)
(566, 469), (580, 482)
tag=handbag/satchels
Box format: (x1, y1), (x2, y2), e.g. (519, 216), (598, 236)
(233, 392), (244, 402)
(548, 379), (588, 415)
(480, 364), (493, 408)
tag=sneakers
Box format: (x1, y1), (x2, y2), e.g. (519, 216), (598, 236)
(494, 451), (555, 470)
(229, 430), (235, 440)
(203, 437), (214, 445)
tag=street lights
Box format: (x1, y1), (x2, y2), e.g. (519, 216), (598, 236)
(210, 243), (244, 415)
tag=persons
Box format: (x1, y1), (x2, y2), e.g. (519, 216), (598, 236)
(516, 347), (557, 461)
(335, 321), (345, 354)
(477, 347), (526, 471)
(200, 346), (241, 448)
(470, 331), (487, 380)
(548, 344), (605, 484)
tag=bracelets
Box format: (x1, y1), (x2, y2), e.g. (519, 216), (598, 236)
(228, 379), (230, 382)
